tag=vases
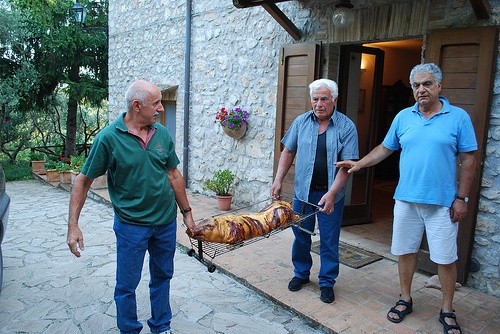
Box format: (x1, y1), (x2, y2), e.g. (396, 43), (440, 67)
(71, 171), (79, 185)
(223, 121), (246, 139)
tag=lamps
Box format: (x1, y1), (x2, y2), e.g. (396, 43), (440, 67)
(333, 0), (355, 27)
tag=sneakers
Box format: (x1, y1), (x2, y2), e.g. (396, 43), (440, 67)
(288, 274), (310, 291)
(320, 285), (335, 303)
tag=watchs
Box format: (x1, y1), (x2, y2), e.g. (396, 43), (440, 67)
(456, 196), (469, 203)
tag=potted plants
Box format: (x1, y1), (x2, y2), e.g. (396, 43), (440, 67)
(58, 163), (71, 183)
(204, 170), (234, 211)
(44, 160), (59, 181)
(29, 152), (46, 172)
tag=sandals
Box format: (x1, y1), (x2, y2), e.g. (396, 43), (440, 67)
(439, 308), (462, 334)
(387, 296), (412, 323)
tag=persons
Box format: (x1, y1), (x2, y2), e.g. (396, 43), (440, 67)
(333, 63), (479, 334)
(271, 78), (360, 302)
(65, 80), (196, 334)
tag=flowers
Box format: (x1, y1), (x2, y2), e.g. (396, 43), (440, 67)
(214, 108), (248, 129)
(70, 153), (86, 172)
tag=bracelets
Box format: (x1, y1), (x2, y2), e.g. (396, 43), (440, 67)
(180, 207), (191, 214)
